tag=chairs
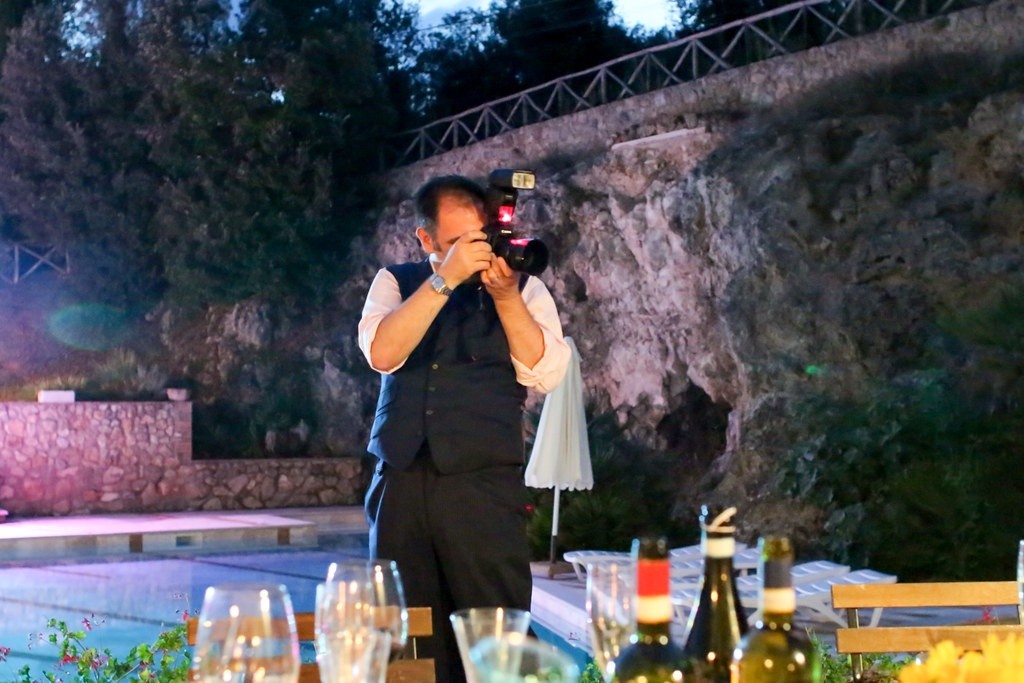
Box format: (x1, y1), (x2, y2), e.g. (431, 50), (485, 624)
(831, 580), (1024, 683)
(184, 606), (439, 683)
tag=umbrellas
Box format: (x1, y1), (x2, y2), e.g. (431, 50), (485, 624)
(523, 335), (594, 579)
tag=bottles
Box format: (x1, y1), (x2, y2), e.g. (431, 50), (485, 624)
(683, 504), (750, 683)
(730, 536), (822, 683)
(612, 533), (688, 683)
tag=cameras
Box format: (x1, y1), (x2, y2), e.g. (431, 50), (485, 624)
(474, 168), (548, 285)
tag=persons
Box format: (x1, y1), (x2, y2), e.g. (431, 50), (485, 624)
(357, 173), (572, 683)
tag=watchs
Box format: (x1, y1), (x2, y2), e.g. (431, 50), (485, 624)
(429, 271), (454, 297)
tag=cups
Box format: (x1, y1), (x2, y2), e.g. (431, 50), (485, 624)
(449, 607), (532, 683)
(470, 633), (581, 683)
(192, 583), (300, 683)
(324, 559), (408, 664)
(313, 581), (393, 683)
